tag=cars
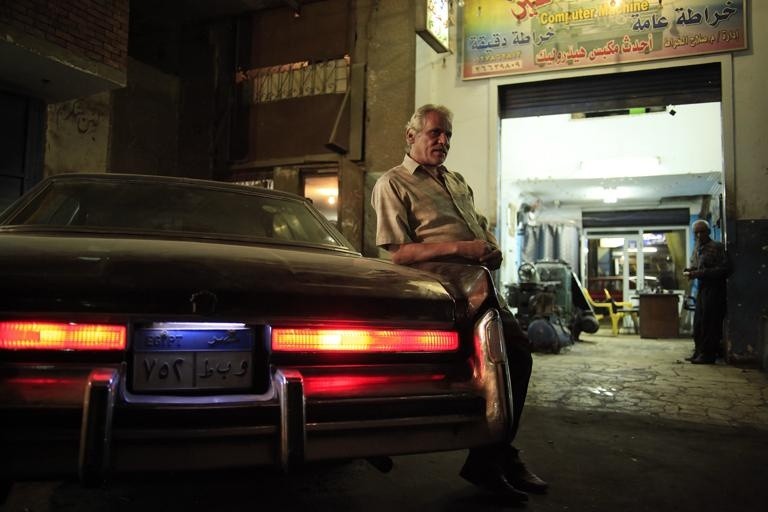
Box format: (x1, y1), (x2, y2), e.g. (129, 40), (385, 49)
(0, 168), (522, 490)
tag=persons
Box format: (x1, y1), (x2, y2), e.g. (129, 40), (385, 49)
(684, 219), (734, 365)
(370, 103), (549, 501)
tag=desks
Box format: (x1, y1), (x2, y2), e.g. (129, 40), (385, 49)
(638, 292), (681, 338)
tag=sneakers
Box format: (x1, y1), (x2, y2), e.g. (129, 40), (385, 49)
(685, 352), (710, 364)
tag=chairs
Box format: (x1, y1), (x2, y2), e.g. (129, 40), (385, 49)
(586, 287), (637, 335)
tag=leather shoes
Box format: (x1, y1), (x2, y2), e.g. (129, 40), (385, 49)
(459, 466), (530, 503)
(506, 463), (548, 493)
